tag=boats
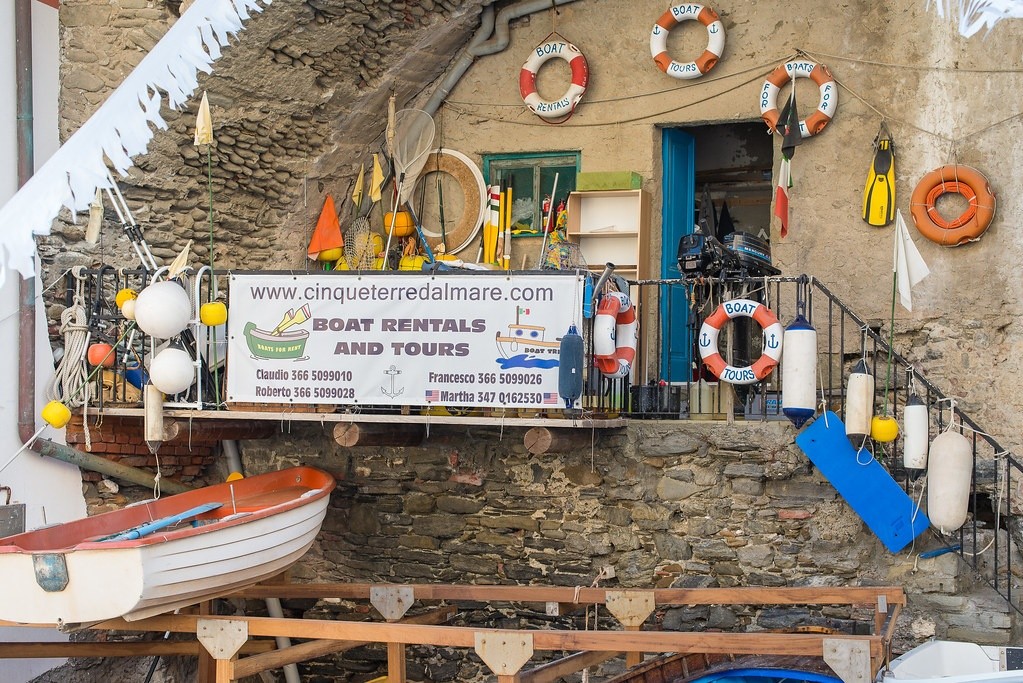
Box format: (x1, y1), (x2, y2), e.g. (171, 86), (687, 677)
(0, 463), (336, 627)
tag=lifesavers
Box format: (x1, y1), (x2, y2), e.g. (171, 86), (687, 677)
(699, 299), (783, 383)
(650, 4), (725, 80)
(519, 41), (587, 117)
(760, 60), (837, 140)
(594, 293), (637, 378)
(909, 163), (997, 248)
(925, 182), (977, 230)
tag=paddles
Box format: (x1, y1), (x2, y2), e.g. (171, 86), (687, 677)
(103, 501), (223, 542)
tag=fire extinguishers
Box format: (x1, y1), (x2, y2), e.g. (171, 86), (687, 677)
(541, 193), (554, 233)
